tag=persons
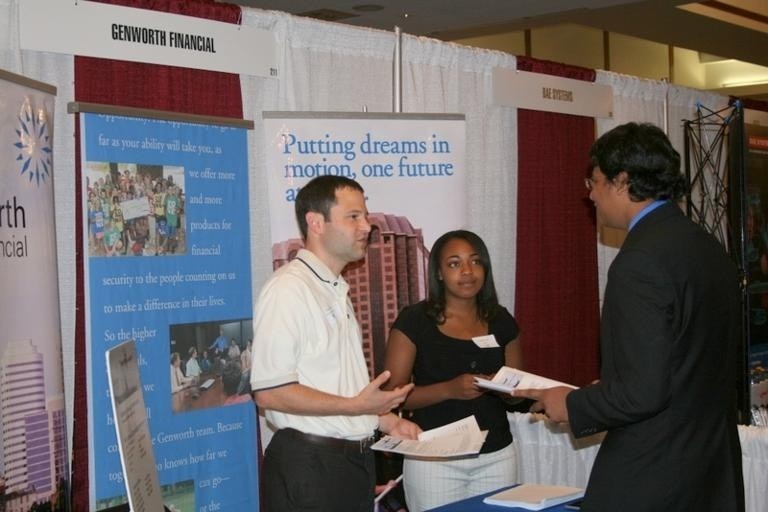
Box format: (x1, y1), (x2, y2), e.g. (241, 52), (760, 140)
(84, 167), (187, 257)
(507, 119), (746, 510)
(169, 328), (254, 406)
(248, 174), (427, 511)
(380, 227), (527, 512)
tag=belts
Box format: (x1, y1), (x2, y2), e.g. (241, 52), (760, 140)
(285, 428), (375, 458)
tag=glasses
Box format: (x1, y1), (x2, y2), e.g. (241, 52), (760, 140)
(584, 175), (618, 190)
